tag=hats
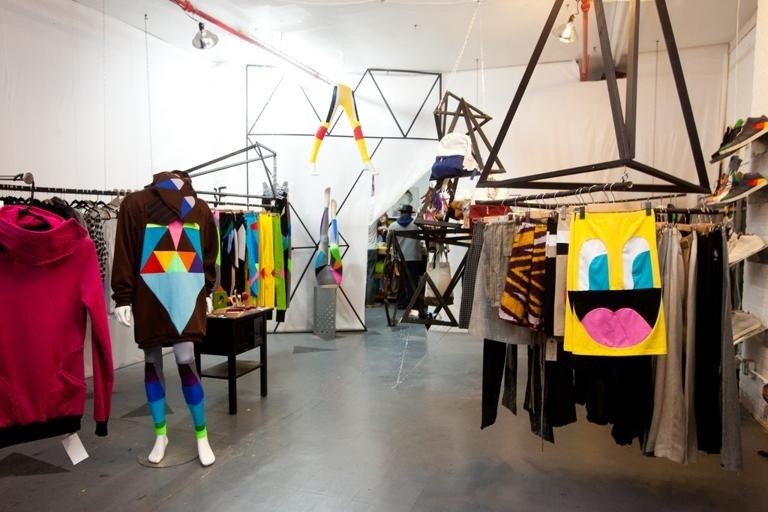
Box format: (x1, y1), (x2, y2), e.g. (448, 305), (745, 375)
(400, 205), (415, 213)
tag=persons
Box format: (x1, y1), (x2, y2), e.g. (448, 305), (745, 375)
(386, 204), (427, 319)
(377, 214), (390, 242)
(110, 171), (220, 467)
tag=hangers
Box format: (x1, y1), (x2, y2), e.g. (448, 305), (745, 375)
(472, 180), (730, 237)
(0, 183), (281, 220)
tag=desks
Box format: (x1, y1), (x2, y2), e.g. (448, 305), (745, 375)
(194, 305), (274, 415)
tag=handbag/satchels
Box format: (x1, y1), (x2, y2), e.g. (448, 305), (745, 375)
(431, 132), (471, 178)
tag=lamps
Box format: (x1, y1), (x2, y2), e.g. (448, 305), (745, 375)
(552, 0), (580, 44)
(184, 1), (219, 50)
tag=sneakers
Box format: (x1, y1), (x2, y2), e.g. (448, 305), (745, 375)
(702, 171), (767, 204)
(711, 115), (768, 154)
(727, 230), (765, 263)
(732, 308), (760, 339)
